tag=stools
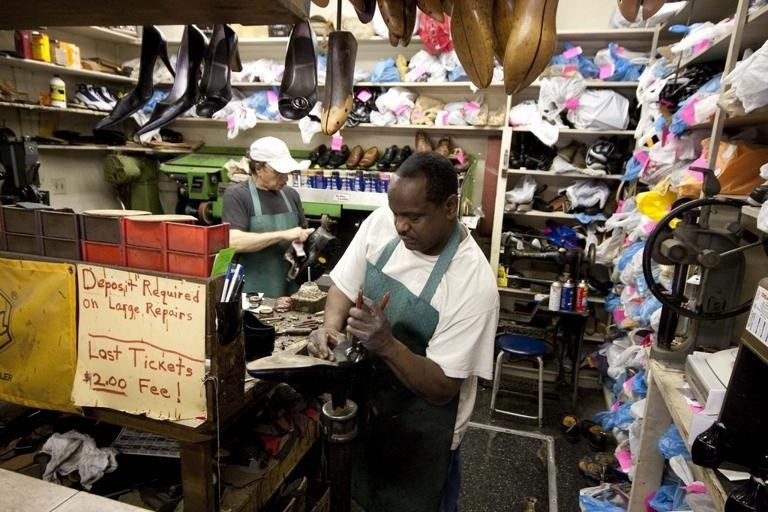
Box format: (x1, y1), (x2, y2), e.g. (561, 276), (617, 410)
(488, 336), (547, 430)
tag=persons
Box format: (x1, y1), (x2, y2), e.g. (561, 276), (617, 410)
(222, 134), (317, 299)
(307, 152), (499, 511)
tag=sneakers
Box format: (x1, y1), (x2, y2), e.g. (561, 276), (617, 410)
(556, 138), (588, 168)
(70, 81), (120, 113)
(293, 226), (337, 287)
(472, 102), (508, 127)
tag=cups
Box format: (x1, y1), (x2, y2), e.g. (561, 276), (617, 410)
(39, 91), (51, 106)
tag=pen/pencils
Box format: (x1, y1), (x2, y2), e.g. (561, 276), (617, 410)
(219, 260), (247, 301)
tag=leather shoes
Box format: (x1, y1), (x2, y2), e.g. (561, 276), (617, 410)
(275, 18), (319, 121)
(503, 193), (535, 213)
(239, 308), (278, 360)
(376, 144), (412, 172)
(241, 347), (370, 403)
(306, 142), (350, 174)
(345, 143), (378, 171)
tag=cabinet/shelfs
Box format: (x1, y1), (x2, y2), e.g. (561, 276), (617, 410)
(1, 26), (161, 115)
(173, 32), (506, 136)
(483, 25), (657, 391)
(602, 3), (766, 510)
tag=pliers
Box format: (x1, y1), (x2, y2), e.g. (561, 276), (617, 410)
(344, 288), (391, 365)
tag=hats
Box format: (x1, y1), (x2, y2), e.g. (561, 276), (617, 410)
(247, 134), (302, 176)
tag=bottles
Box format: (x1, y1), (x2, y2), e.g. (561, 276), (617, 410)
(32, 31), (59, 64)
(13, 29), (32, 61)
(294, 171), (391, 194)
(550, 276), (592, 311)
(498, 263), (509, 288)
(50, 74), (67, 109)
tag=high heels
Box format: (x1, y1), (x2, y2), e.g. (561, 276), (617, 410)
(132, 22), (210, 137)
(94, 21), (178, 131)
(195, 21), (244, 118)
